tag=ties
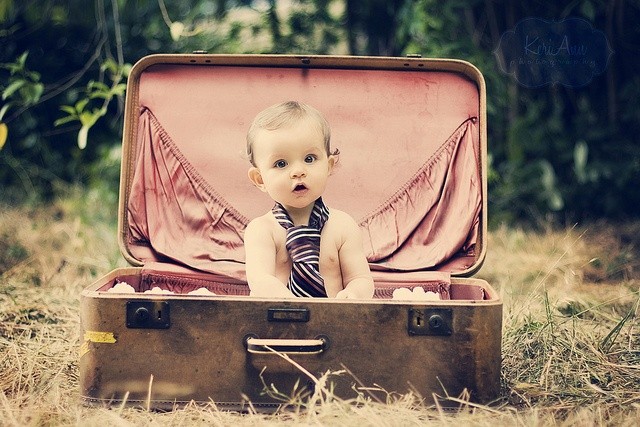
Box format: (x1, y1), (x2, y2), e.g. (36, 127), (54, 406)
(272, 195), (330, 298)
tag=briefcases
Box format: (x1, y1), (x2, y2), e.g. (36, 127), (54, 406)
(82, 50), (502, 410)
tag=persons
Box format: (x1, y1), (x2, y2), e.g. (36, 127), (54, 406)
(243, 100), (376, 304)
